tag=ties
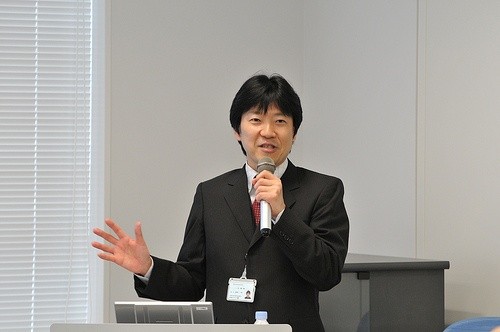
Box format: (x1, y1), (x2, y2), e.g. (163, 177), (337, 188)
(252, 198), (260, 224)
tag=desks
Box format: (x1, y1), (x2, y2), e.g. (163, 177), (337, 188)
(320, 254), (450, 332)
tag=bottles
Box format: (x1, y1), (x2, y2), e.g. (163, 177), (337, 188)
(253, 310), (268, 326)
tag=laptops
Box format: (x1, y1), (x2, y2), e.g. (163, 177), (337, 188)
(114, 301), (214, 324)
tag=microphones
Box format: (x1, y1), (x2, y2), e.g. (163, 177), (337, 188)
(256, 157), (276, 236)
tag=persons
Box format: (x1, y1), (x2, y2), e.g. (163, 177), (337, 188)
(92, 73), (350, 332)
